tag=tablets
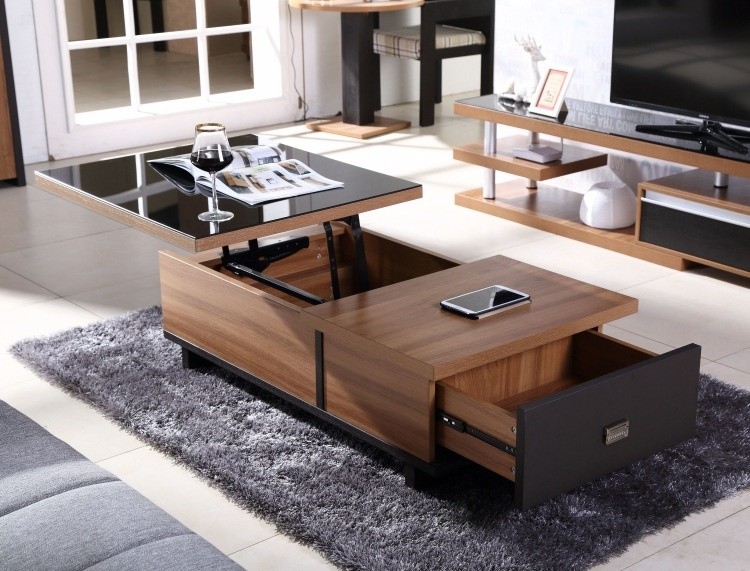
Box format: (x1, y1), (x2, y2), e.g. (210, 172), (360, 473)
(441, 284), (531, 316)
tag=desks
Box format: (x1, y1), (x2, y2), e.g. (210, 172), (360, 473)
(288, 0), (425, 140)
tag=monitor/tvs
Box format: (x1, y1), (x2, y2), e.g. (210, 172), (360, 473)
(609, 0), (750, 153)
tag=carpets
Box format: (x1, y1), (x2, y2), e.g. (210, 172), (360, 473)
(8, 306), (750, 570)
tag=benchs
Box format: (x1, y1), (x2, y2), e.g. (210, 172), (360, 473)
(1, 401), (244, 571)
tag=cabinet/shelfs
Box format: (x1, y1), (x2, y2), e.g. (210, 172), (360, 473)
(452, 95), (750, 278)
(34, 133), (701, 511)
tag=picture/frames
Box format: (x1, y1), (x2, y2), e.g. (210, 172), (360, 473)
(529, 64), (575, 118)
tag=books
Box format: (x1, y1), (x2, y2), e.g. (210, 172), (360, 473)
(144, 143), (344, 206)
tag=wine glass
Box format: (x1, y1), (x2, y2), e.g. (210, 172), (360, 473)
(191, 123), (235, 222)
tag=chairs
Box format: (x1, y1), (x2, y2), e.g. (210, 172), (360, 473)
(373, 0), (495, 127)
